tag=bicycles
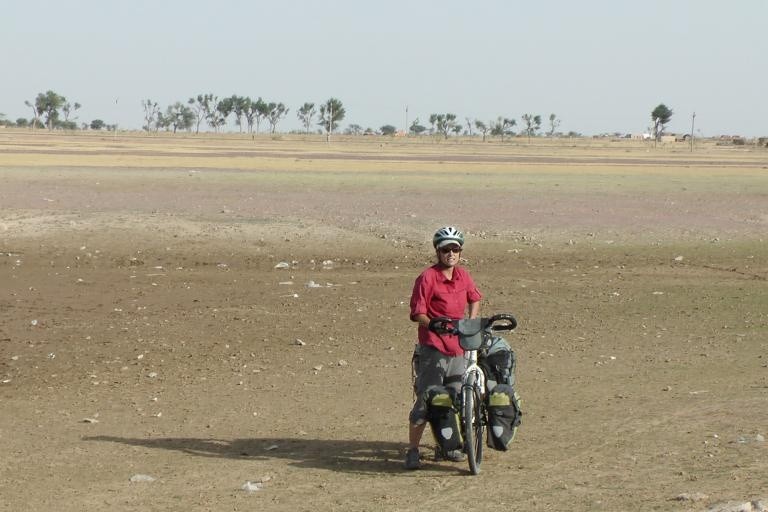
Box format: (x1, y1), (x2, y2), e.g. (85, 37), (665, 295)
(428, 314), (517, 476)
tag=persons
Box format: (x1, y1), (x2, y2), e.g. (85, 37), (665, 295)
(405, 225), (482, 469)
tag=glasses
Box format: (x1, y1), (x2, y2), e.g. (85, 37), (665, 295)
(441, 247), (460, 253)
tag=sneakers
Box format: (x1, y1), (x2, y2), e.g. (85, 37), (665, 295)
(403, 448), (423, 471)
(433, 447), (467, 462)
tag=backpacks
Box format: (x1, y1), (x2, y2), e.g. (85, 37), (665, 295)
(484, 384), (522, 451)
(426, 385), (466, 453)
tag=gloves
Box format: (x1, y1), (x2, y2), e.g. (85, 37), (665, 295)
(431, 316), (451, 333)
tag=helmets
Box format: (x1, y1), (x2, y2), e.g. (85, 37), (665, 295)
(433, 226), (465, 247)
